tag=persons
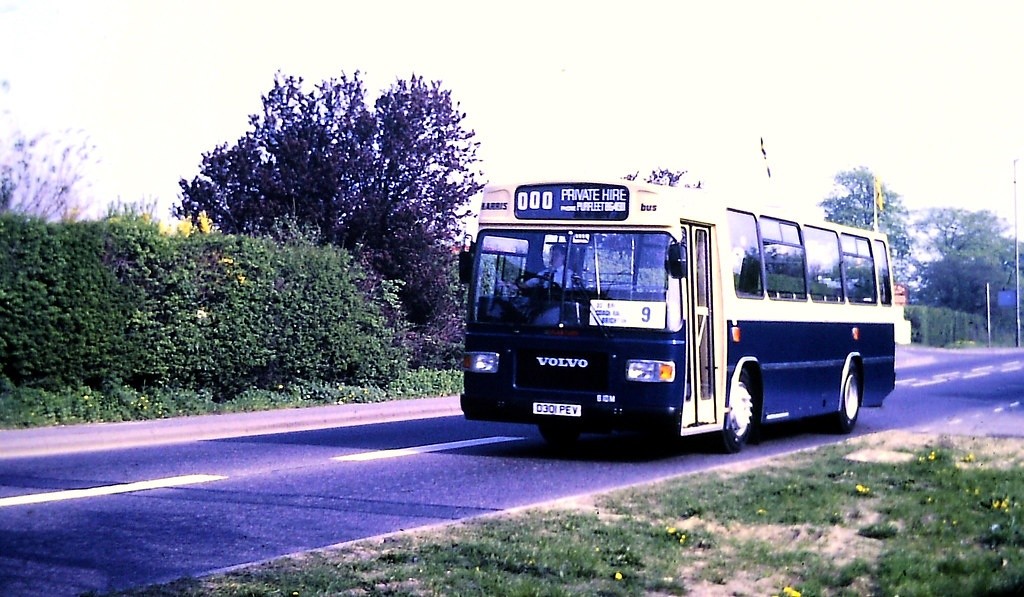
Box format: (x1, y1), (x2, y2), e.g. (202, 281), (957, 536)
(518, 244), (574, 289)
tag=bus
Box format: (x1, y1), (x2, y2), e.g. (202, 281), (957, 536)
(457, 176), (897, 451)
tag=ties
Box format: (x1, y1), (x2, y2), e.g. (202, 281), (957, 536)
(550, 270), (558, 282)
